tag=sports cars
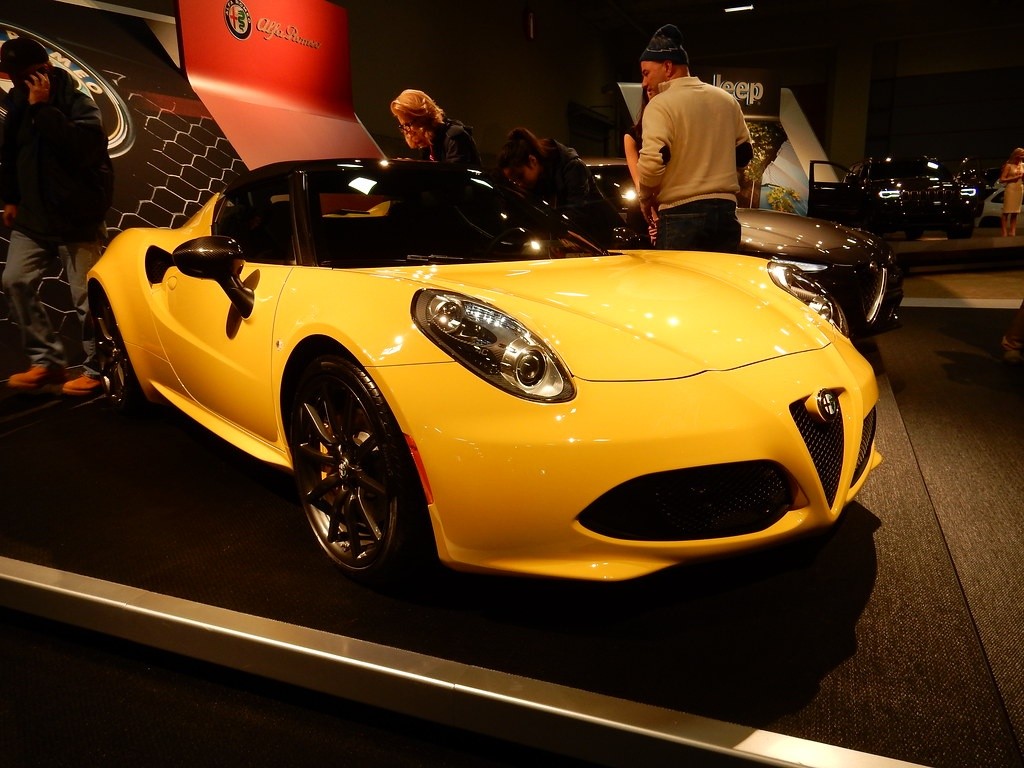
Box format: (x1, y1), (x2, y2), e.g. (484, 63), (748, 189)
(580, 155), (907, 342)
(83, 156), (881, 589)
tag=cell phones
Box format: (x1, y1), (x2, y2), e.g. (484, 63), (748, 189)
(25, 66), (51, 89)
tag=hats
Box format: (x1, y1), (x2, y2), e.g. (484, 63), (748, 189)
(639, 23), (688, 64)
(0, 38), (49, 74)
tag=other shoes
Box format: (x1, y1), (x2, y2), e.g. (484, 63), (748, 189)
(61, 375), (106, 397)
(7, 363), (73, 391)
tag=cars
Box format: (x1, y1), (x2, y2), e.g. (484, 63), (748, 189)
(807, 150), (1024, 242)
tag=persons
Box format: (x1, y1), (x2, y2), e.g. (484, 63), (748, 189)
(638, 23), (752, 253)
(498, 128), (626, 249)
(390, 89), (480, 164)
(1001, 301), (1024, 363)
(624, 86), (653, 249)
(0, 38), (114, 395)
(999, 148), (1024, 237)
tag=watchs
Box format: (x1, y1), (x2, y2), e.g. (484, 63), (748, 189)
(638, 193), (655, 202)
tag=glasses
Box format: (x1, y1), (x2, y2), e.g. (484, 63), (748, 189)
(397, 117), (420, 132)
